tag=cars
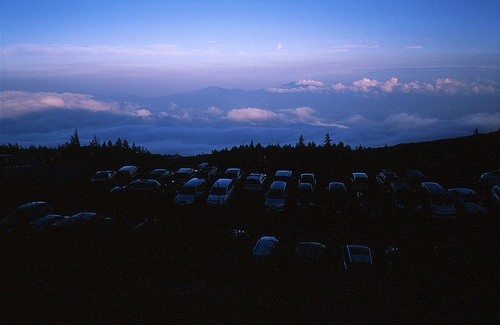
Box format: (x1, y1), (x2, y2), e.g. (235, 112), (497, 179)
(250, 235), (284, 267)
(295, 239), (328, 267)
(220, 167), (245, 186)
(3, 201), (114, 238)
(89, 170), (114, 188)
(111, 166), (139, 184)
(146, 168), (174, 185)
(207, 178), (236, 208)
(265, 169), (493, 222)
(111, 179), (164, 193)
(243, 172), (268, 191)
(172, 177), (209, 209)
(340, 244), (373, 270)
(195, 162), (219, 180)
(173, 168), (195, 186)
